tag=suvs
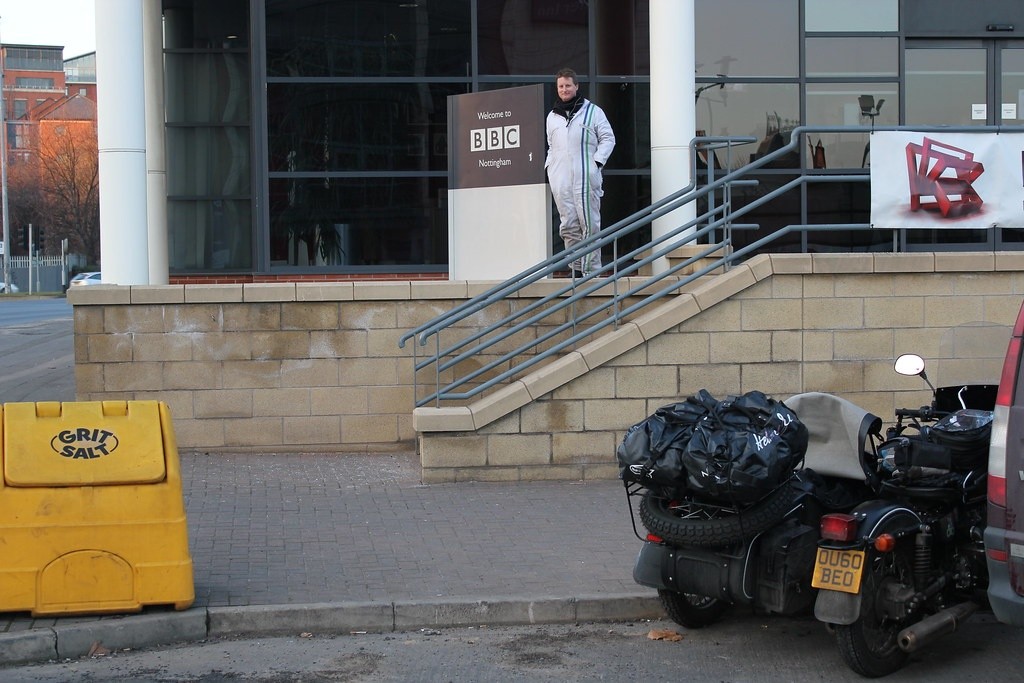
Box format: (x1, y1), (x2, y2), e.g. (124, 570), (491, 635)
(69, 273), (101, 287)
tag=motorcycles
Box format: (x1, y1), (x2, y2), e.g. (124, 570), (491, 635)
(622, 351), (1001, 679)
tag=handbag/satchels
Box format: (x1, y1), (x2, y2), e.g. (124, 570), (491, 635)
(618, 388), (810, 506)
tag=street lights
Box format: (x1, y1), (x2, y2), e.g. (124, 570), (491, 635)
(859, 92), (884, 133)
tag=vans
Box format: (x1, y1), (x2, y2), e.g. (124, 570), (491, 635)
(981, 292), (1023, 635)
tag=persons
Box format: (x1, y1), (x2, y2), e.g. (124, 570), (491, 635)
(543, 69), (616, 278)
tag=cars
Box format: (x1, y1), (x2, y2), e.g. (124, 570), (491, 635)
(0, 283), (19, 295)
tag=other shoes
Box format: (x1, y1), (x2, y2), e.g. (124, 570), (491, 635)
(564, 270), (583, 277)
